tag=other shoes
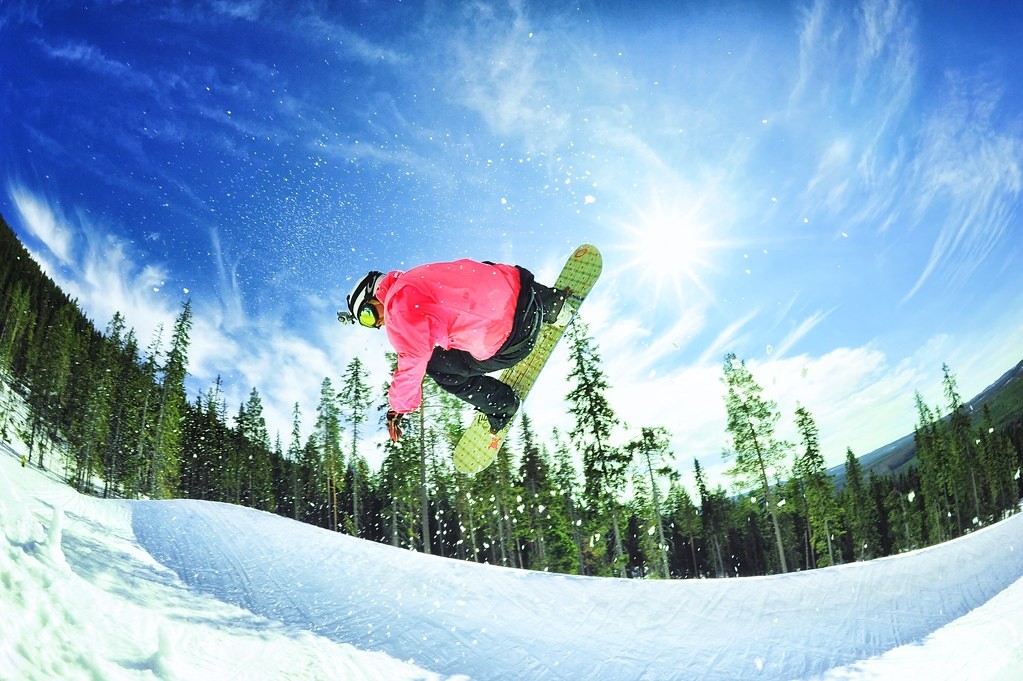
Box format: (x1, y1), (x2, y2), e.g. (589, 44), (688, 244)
(513, 396), (522, 414)
(556, 309), (572, 327)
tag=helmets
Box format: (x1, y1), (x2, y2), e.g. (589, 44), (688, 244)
(347, 270), (388, 321)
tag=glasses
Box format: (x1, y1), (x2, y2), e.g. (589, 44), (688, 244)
(360, 303), (381, 329)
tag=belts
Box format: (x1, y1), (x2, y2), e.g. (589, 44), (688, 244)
(501, 296), (539, 356)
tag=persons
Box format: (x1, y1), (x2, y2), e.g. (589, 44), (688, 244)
(348, 258), (569, 443)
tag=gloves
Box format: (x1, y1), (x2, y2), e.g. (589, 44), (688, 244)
(387, 408), (404, 443)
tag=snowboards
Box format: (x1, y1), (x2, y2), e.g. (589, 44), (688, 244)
(451, 241), (602, 474)
